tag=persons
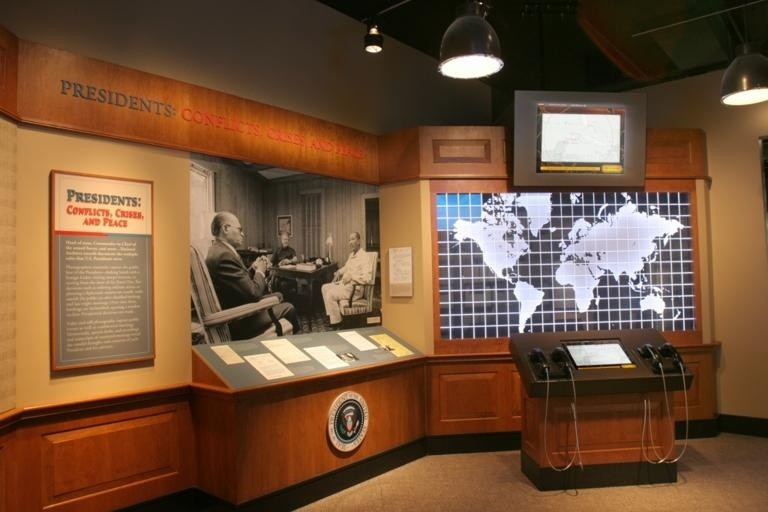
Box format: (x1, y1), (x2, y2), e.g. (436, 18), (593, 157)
(205, 211), (301, 341)
(267, 233), (298, 292)
(321, 232), (372, 331)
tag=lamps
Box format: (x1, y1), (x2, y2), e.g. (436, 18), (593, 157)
(365, 14), (384, 53)
(721, 7), (767, 108)
(437, 1), (505, 82)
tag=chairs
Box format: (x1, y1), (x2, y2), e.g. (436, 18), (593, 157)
(187, 244), (294, 345)
(334, 251), (378, 326)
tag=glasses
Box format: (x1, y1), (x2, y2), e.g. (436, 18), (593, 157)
(224, 224), (243, 232)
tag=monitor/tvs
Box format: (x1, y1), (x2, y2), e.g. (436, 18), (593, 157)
(514, 89), (648, 188)
(562, 338), (636, 369)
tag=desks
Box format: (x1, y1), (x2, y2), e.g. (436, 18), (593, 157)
(266, 261), (337, 321)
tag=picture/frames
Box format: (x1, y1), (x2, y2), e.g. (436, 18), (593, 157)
(47, 168), (157, 372)
(276, 215), (293, 237)
